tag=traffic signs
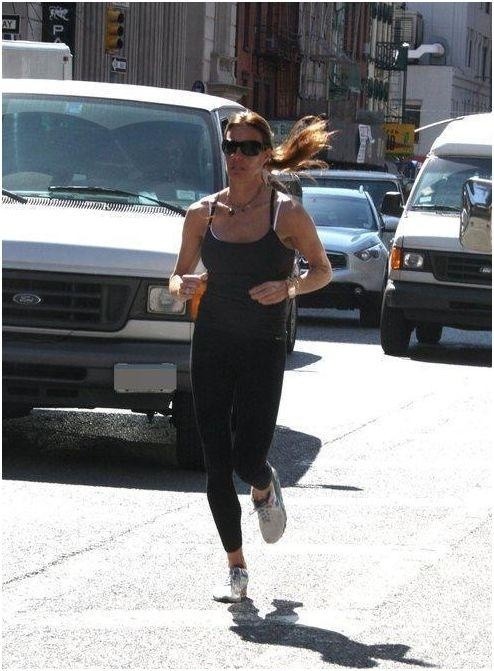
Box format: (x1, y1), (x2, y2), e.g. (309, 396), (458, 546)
(109, 56), (127, 74)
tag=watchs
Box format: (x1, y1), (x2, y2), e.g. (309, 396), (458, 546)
(287, 278), (296, 299)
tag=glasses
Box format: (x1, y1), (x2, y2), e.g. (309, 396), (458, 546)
(222, 140), (271, 157)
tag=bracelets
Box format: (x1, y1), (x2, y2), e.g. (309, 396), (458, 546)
(295, 277), (304, 295)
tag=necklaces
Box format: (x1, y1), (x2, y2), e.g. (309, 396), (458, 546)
(227, 183), (263, 217)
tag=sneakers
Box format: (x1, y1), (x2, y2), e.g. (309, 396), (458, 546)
(213, 568), (249, 603)
(250, 466), (287, 544)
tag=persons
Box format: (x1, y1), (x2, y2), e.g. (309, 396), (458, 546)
(169, 111), (333, 603)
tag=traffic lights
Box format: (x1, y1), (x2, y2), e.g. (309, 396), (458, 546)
(104, 7), (124, 52)
